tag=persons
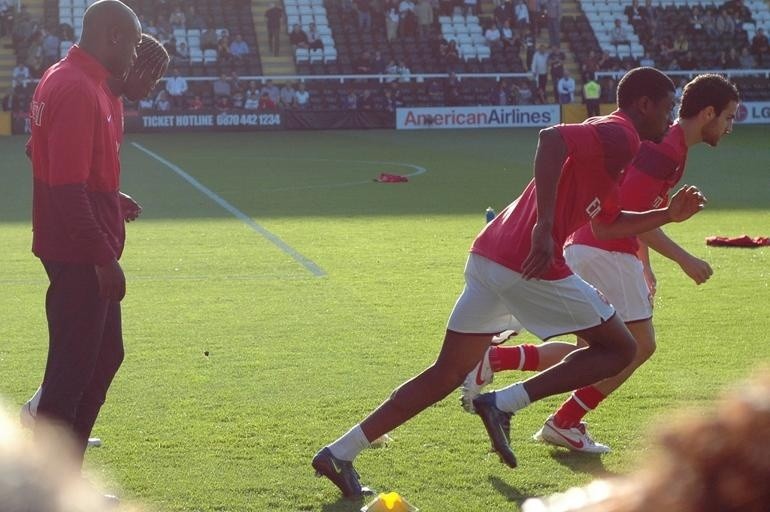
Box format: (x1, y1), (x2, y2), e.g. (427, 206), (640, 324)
(454, 72), (742, 458)
(24, 0), (144, 512)
(24, 34), (170, 449)
(309, 66), (708, 503)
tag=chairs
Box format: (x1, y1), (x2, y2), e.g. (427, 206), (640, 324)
(560, 0), (768, 104)
(285, 1), (528, 75)
(132, 1), (266, 89)
(44, 1), (91, 67)
(303, 79), (542, 107)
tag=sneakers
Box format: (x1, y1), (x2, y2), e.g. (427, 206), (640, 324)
(311, 446), (362, 496)
(18, 400), (103, 447)
(542, 414), (611, 453)
(459, 347), (517, 468)
